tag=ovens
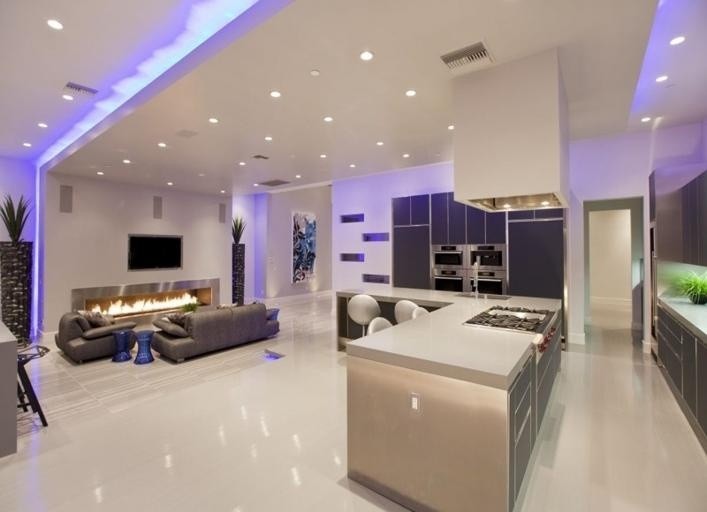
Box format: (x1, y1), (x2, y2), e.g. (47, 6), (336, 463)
(467, 244), (507, 294)
(430, 245), (467, 292)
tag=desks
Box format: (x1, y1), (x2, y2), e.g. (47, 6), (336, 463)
(0, 323), (17, 457)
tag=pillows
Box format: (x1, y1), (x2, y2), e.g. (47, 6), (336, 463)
(78, 306), (111, 327)
(165, 312), (184, 326)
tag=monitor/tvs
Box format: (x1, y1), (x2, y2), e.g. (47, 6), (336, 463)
(128, 233), (183, 270)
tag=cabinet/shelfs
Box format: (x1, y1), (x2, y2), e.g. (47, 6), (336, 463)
(538, 309), (561, 432)
(345, 322), (538, 512)
(655, 297), (706, 456)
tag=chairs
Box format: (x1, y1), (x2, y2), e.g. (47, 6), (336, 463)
(346, 293), (429, 338)
(54, 308), (137, 364)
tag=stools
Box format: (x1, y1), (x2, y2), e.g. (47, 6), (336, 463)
(133, 330), (154, 364)
(112, 328), (133, 363)
(13, 337), (50, 427)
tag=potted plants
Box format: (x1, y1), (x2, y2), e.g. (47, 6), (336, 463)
(228, 214), (247, 305)
(678, 273), (707, 304)
(0, 187), (35, 347)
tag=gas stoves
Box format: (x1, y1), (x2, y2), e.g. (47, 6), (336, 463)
(461, 304), (561, 354)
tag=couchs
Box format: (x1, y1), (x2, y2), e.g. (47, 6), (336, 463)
(150, 303), (279, 364)
